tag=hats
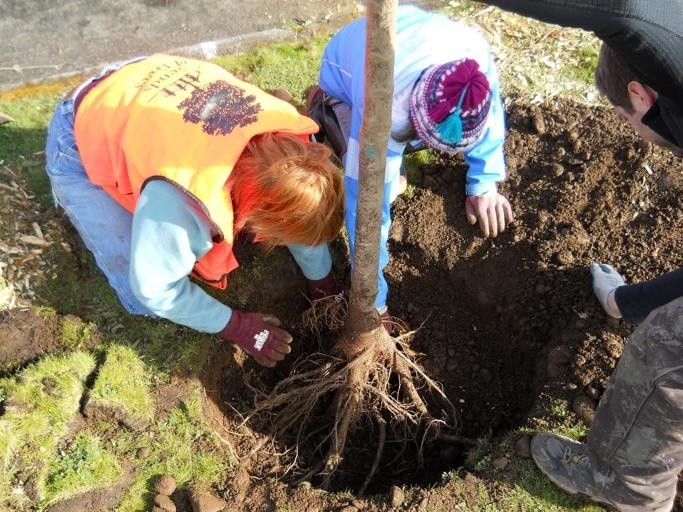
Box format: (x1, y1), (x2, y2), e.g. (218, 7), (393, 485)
(407, 59), (493, 157)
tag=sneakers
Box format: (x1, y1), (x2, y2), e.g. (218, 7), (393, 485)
(532, 430), (679, 512)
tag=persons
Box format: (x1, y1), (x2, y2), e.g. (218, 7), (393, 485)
(474, 0), (683, 510)
(44, 53), (344, 369)
(305, 5), (514, 336)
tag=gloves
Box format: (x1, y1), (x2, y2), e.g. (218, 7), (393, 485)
(306, 272), (337, 302)
(590, 260), (629, 321)
(219, 309), (294, 369)
(376, 305), (393, 332)
(465, 190), (515, 237)
